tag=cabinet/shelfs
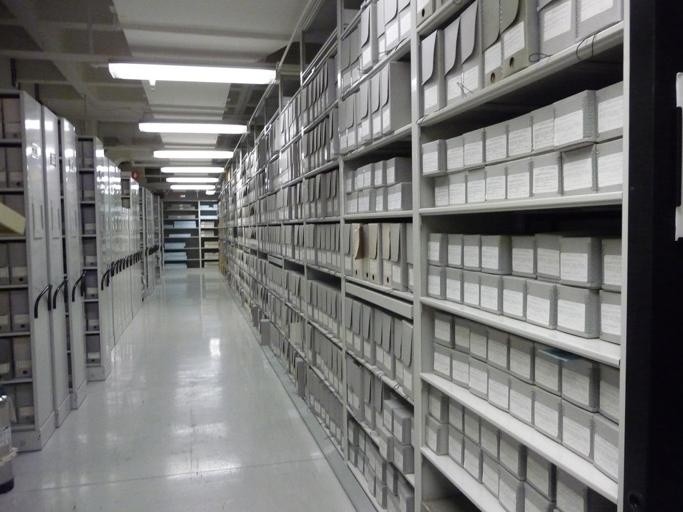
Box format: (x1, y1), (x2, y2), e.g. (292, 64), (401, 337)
(217, 1), (683, 511)
(1, 87), (166, 454)
(161, 200), (219, 268)
